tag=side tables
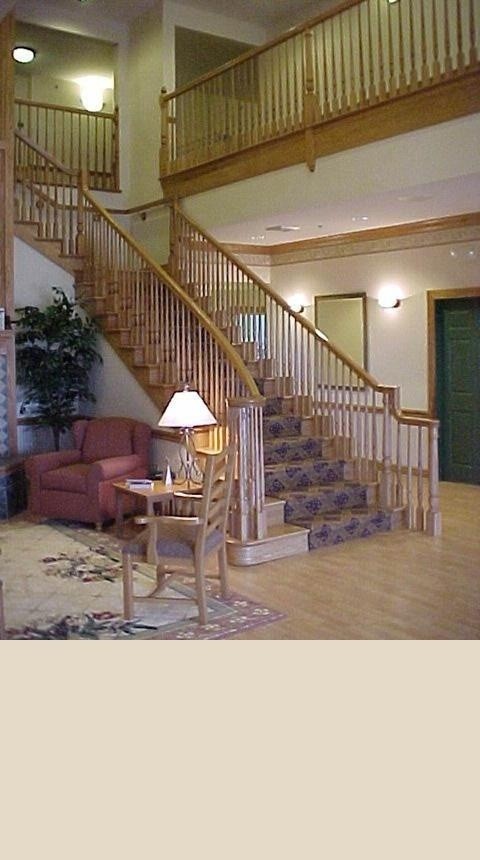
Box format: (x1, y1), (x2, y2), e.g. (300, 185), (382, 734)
(112, 478), (202, 539)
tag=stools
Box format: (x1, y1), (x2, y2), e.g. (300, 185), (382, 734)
(0, 456), (27, 518)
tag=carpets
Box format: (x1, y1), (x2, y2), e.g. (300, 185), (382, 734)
(0, 509), (288, 640)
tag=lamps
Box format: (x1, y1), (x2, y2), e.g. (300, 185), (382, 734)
(78, 77), (106, 113)
(378, 288), (399, 309)
(289, 295), (310, 313)
(158, 385), (218, 488)
(11, 47), (36, 64)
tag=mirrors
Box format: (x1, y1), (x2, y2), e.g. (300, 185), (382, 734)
(314, 292), (367, 391)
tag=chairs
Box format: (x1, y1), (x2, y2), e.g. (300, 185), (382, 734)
(122, 444), (237, 626)
(25, 416), (151, 531)
